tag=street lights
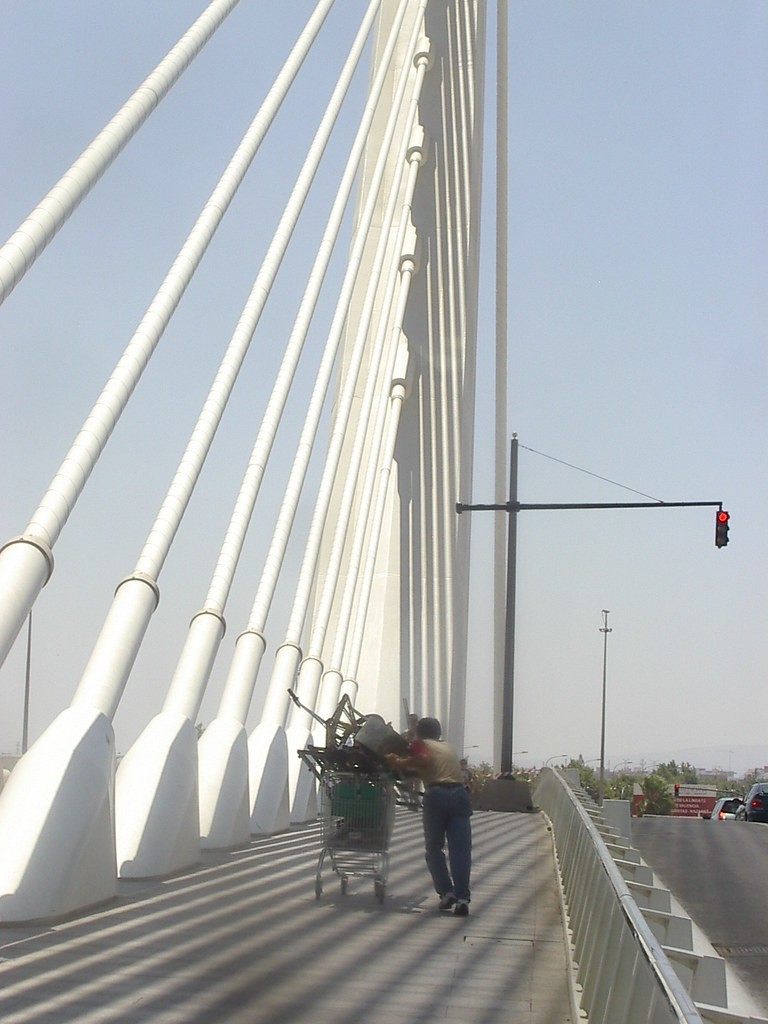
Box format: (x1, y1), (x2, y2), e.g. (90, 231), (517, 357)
(613, 761), (633, 778)
(599, 608), (613, 806)
(584, 758), (602, 767)
(546, 754), (568, 769)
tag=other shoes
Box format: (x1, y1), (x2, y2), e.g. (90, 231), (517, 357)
(438, 892), (457, 910)
(455, 898), (469, 915)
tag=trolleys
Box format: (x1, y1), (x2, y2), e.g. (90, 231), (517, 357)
(308, 769), (397, 906)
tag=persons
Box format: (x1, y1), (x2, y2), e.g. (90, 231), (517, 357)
(400, 714), (421, 812)
(460, 758), (479, 816)
(384, 718), (474, 916)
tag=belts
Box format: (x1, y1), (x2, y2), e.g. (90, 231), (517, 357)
(424, 780), (464, 789)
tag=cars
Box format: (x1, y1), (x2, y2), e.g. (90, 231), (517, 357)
(703, 798), (743, 821)
(733, 781), (768, 822)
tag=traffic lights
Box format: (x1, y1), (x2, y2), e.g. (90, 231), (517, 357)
(716, 509), (730, 551)
(674, 784), (682, 798)
